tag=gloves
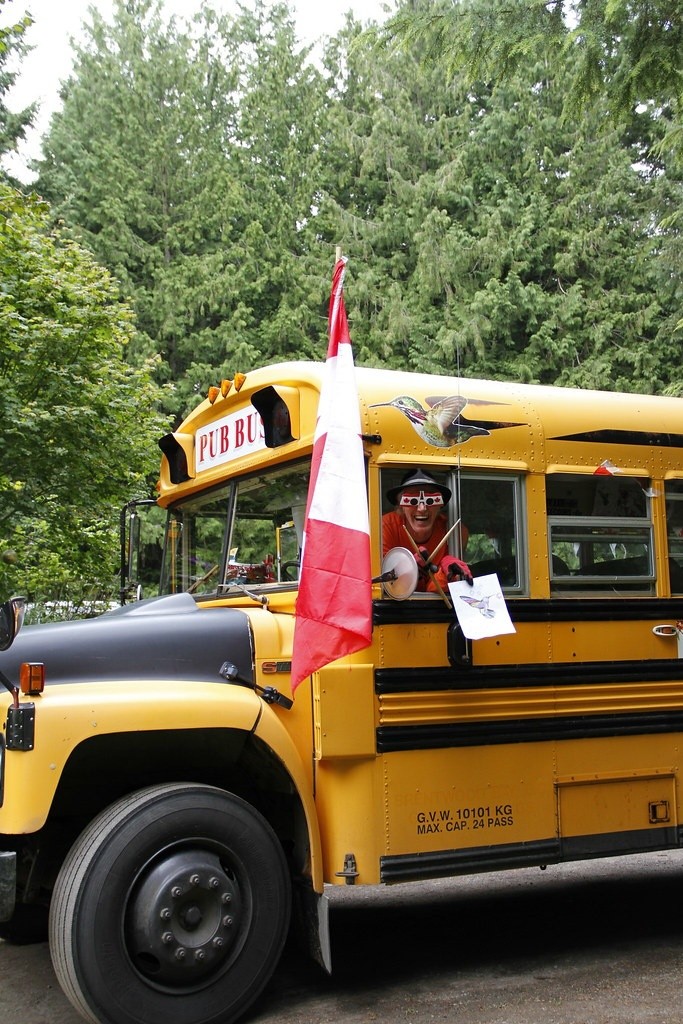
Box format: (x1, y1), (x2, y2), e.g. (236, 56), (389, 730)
(442, 555), (473, 587)
(413, 546), (438, 581)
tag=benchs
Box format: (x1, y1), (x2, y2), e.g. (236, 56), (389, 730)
(467, 556), (683, 597)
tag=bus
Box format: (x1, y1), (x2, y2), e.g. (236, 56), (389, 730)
(0, 360), (683, 1024)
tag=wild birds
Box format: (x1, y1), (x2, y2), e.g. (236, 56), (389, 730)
(370, 396), (529, 447)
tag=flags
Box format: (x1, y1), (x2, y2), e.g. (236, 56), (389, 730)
(289, 255), (374, 701)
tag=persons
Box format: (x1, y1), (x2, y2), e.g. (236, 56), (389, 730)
(382, 468), (474, 593)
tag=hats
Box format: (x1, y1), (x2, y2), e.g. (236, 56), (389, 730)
(386, 468), (452, 509)
